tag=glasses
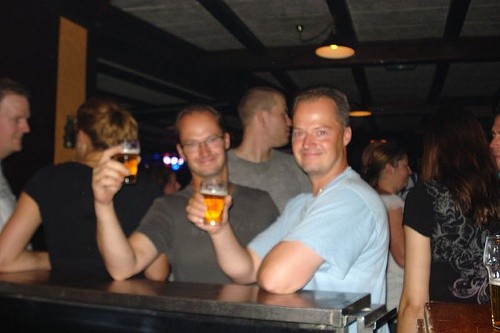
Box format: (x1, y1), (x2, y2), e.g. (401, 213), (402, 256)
(182, 134), (225, 151)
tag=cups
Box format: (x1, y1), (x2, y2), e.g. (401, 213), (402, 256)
(116, 139), (140, 182)
(200, 180), (228, 226)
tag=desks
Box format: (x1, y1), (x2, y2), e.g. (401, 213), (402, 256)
(0, 270), (398, 333)
(425, 301), (500, 333)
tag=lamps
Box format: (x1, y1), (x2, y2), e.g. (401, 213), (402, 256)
(315, 19), (356, 60)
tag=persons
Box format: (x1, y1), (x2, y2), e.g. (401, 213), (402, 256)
(0, 85), (500, 333)
(92, 106), (281, 284)
(186, 88), (390, 333)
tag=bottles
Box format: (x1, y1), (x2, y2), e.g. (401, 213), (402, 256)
(483, 235), (500, 330)
